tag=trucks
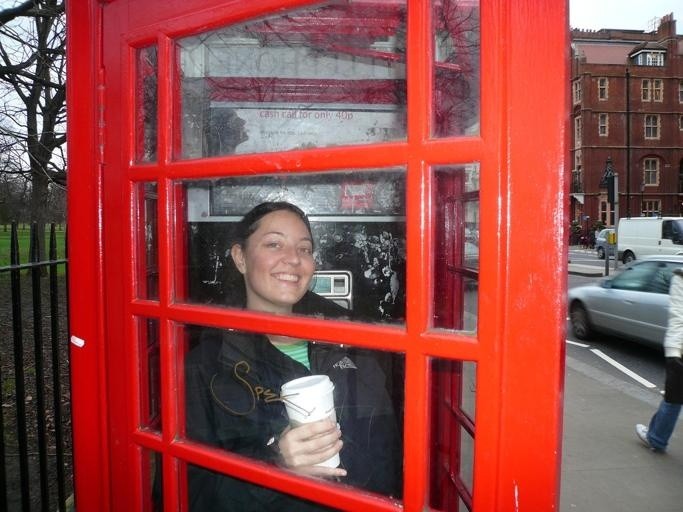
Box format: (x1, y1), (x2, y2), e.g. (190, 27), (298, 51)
(616, 216), (683, 263)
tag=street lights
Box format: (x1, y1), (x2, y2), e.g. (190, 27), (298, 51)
(639, 181), (645, 216)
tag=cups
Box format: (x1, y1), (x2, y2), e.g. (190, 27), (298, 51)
(280, 373), (342, 472)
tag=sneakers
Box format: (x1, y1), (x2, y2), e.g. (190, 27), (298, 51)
(636, 423), (666, 453)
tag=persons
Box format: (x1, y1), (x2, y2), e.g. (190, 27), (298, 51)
(186, 202), (401, 512)
(637, 268), (683, 451)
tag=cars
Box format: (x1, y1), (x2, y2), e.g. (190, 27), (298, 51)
(464, 241), (480, 289)
(564, 254), (682, 358)
(595, 228), (615, 259)
